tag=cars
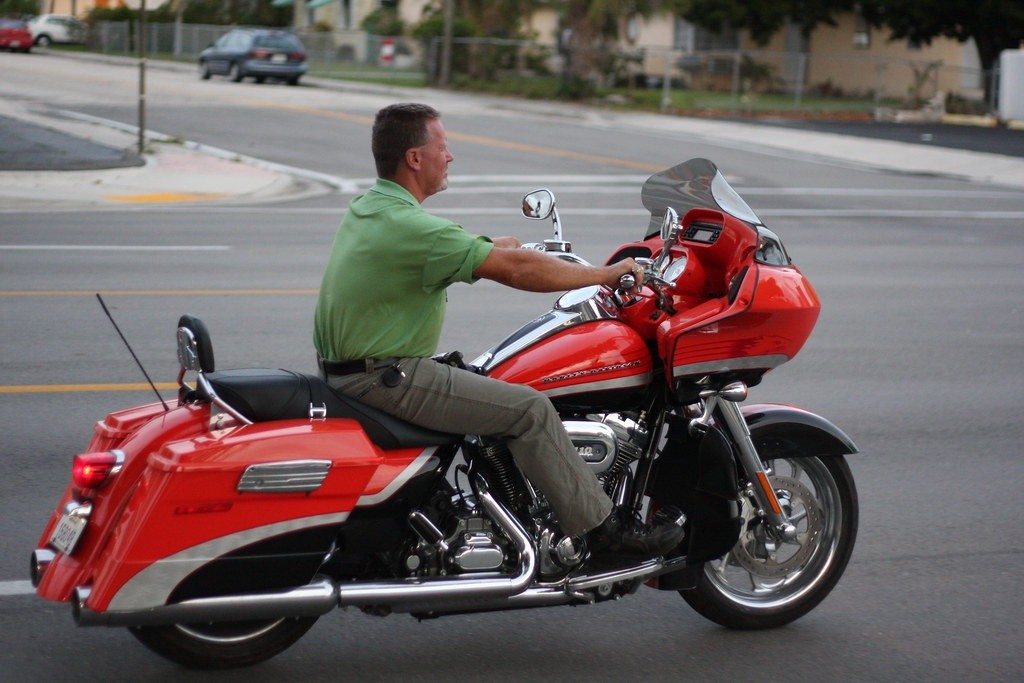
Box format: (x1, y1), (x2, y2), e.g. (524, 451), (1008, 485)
(26, 13), (88, 47)
(197, 28), (309, 86)
(0, 18), (32, 52)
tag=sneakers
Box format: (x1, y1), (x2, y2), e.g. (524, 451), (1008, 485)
(587, 504), (685, 561)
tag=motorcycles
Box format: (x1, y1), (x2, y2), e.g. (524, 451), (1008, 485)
(29, 157), (860, 669)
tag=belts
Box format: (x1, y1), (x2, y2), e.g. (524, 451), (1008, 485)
(316, 352), (401, 375)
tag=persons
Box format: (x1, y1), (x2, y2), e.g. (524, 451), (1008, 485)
(312, 102), (684, 577)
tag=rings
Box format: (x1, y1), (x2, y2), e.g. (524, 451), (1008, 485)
(632, 263), (643, 274)
